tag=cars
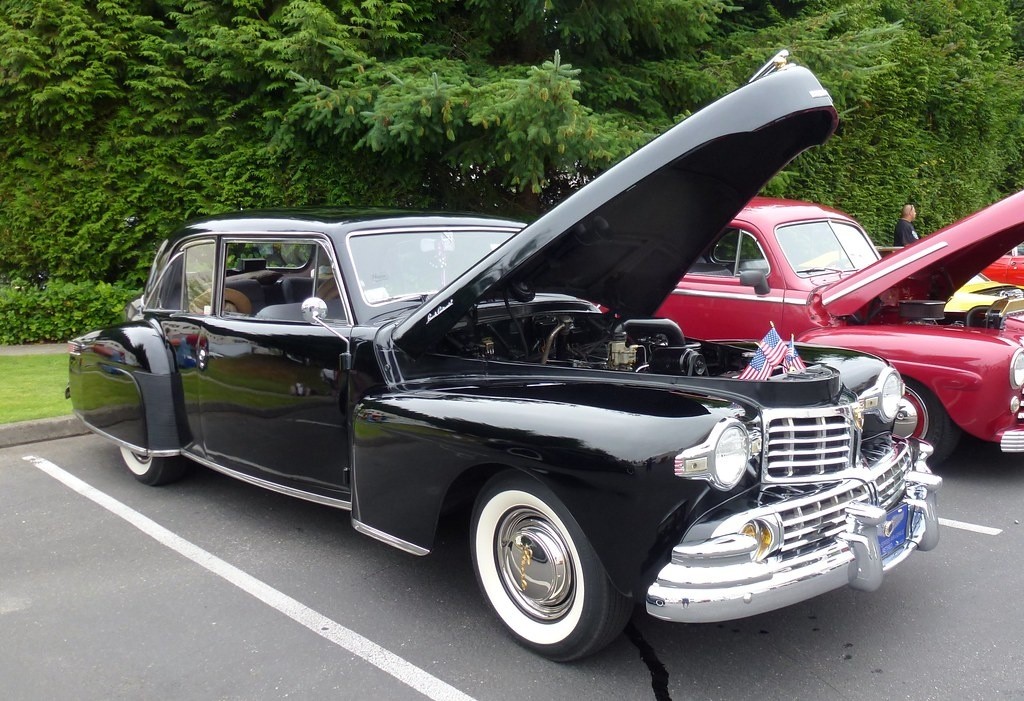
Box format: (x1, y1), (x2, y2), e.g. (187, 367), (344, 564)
(66, 48), (944, 667)
(655, 184), (1023, 467)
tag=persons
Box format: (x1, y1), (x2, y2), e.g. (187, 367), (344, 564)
(165, 324), (208, 374)
(893, 204), (920, 247)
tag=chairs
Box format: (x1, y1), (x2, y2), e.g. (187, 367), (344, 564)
(224, 277), (329, 317)
(730, 259), (769, 275)
(687, 263), (733, 276)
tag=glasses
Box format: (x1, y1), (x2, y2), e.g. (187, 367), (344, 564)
(911, 204), (914, 210)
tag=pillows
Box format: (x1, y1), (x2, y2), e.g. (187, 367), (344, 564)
(226, 270), (284, 285)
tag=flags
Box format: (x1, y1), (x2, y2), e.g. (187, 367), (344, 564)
(783, 342), (807, 374)
(760, 328), (789, 368)
(738, 347), (773, 381)
